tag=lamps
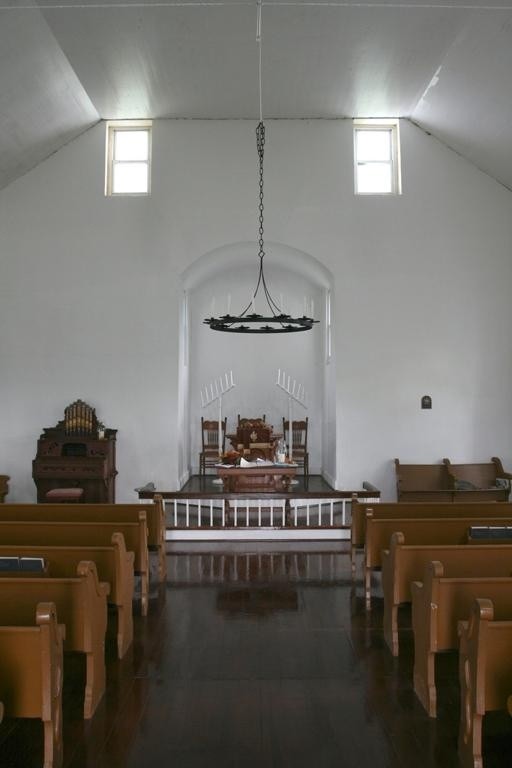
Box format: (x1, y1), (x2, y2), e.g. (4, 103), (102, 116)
(201, 0), (322, 334)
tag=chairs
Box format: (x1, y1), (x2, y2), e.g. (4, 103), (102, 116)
(195, 409), (313, 492)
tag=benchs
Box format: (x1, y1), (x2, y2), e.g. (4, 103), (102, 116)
(395, 457), (512, 502)
(0, 503), (166, 660)
(0, 561), (111, 719)
(0, 602), (66, 768)
(352, 494), (512, 657)
(456, 597), (512, 768)
(410, 560), (512, 717)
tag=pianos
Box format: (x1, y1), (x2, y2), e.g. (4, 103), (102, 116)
(33, 430), (117, 502)
